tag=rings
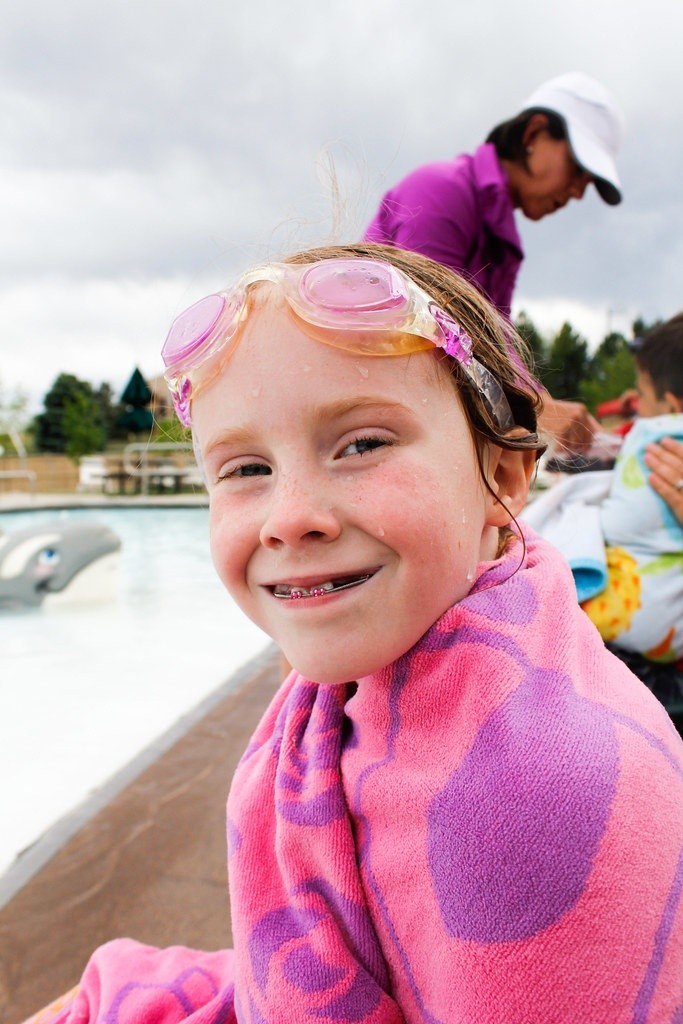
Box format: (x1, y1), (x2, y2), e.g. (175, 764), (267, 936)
(676, 479), (683, 490)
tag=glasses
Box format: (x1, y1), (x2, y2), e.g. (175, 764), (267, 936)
(161, 256), (514, 430)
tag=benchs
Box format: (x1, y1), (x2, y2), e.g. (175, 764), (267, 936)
(106, 472), (141, 493)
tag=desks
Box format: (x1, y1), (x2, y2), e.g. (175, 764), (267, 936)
(108, 457), (182, 493)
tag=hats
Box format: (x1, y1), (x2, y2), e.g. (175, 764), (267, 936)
(522, 72), (624, 206)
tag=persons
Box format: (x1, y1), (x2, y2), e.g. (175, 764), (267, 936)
(594, 366), (641, 440)
(639, 435), (683, 524)
(515, 308), (683, 667)
(355, 69), (628, 461)
(15, 154), (683, 1022)
